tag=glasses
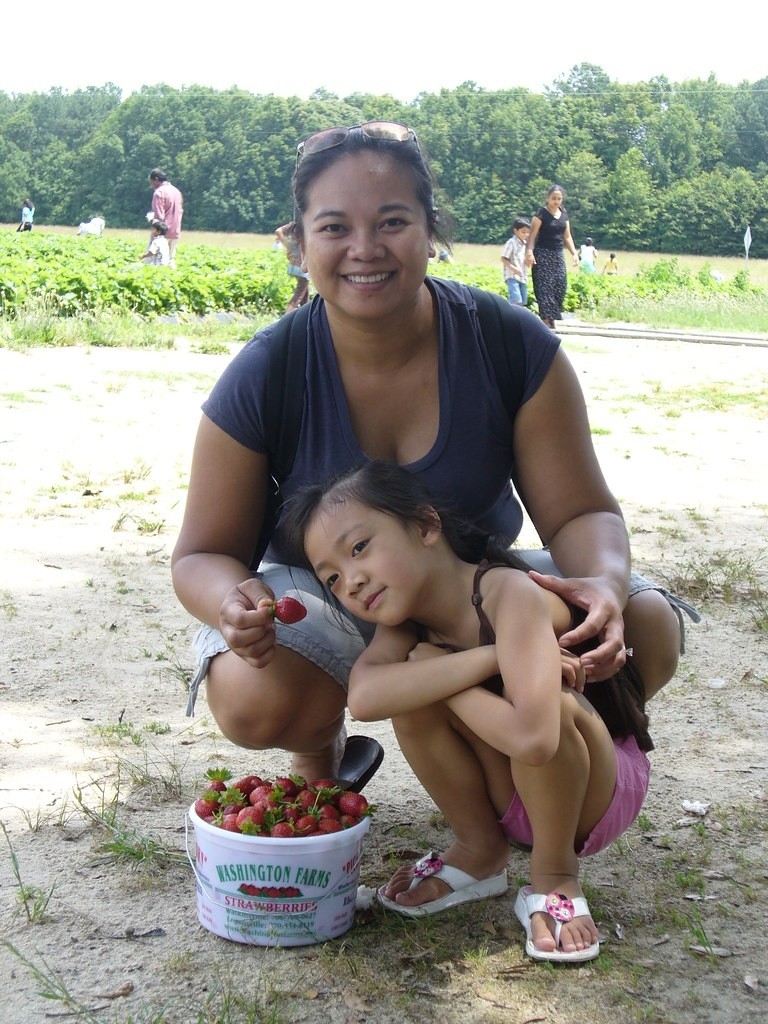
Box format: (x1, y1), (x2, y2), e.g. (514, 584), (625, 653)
(293, 121), (428, 194)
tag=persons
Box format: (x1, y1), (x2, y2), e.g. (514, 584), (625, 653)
(145, 168), (184, 269)
(139, 222), (170, 266)
(437, 248), (449, 263)
(281, 460), (654, 962)
(525, 185), (579, 331)
(501, 217), (532, 307)
(601, 252), (618, 276)
(87, 215), (106, 235)
(16, 199), (35, 232)
(578, 237), (598, 269)
(169, 122), (701, 795)
(275, 222), (310, 316)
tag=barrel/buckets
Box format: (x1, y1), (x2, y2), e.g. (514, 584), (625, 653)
(185, 801), (377, 947)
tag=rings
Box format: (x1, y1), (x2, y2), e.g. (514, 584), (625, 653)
(625, 647), (633, 656)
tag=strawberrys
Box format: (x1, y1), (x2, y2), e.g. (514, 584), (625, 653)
(274, 597), (308, 624)
(195, 767), (379, 838)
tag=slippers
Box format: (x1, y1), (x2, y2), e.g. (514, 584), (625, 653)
(514, 885), (600, 963)
(310, 734), (385, 794)
(376, 850), (509, 918)
